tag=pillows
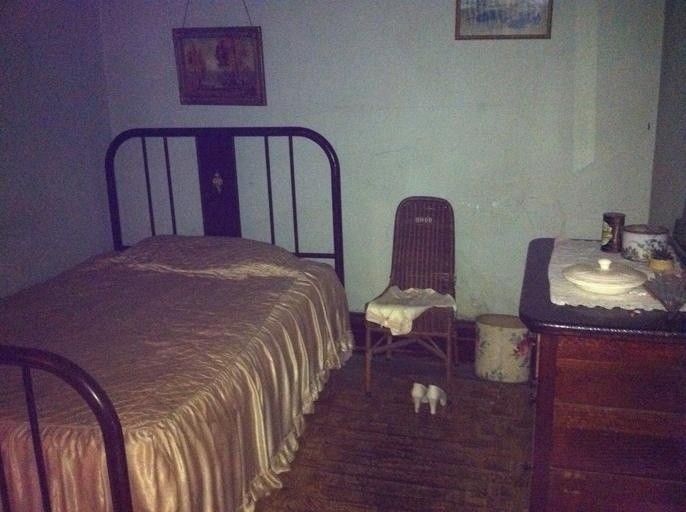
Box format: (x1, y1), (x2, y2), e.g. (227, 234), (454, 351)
(118, 233), (301, 282)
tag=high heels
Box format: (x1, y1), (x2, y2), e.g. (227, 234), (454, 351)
(411, 382), (447, 415)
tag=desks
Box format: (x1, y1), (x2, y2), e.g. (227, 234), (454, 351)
(519, 236), (686, 509)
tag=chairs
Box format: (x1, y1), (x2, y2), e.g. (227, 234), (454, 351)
(364, 196), (459, 404)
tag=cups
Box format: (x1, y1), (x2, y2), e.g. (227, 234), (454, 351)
(601, 212), (626, 253)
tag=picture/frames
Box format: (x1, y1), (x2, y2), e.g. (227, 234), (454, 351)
(172, 27), (267, 106)
(455, 1), (552, 40)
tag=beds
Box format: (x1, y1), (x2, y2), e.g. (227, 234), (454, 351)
(1, 126), (354, 512)
